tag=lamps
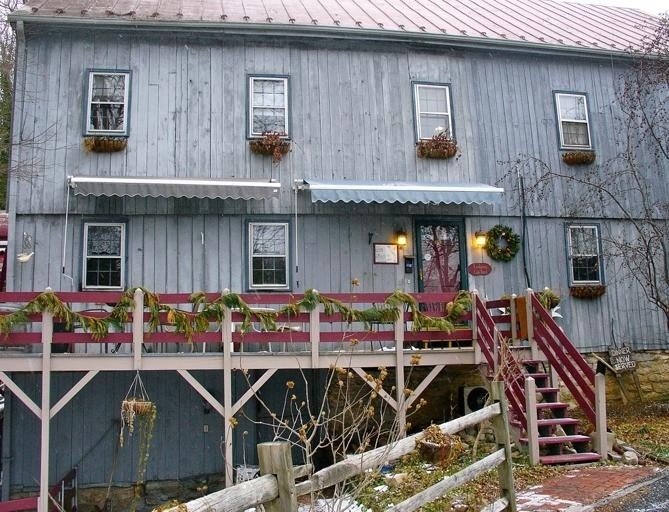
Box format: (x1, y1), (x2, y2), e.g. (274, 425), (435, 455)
(396, 227), (407, 249)
(472, 228), (487, 249)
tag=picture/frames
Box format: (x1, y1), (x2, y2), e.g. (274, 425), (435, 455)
(373, 242), (399, 264)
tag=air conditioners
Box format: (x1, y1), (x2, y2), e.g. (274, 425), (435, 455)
(464, 385), (490, 415)
